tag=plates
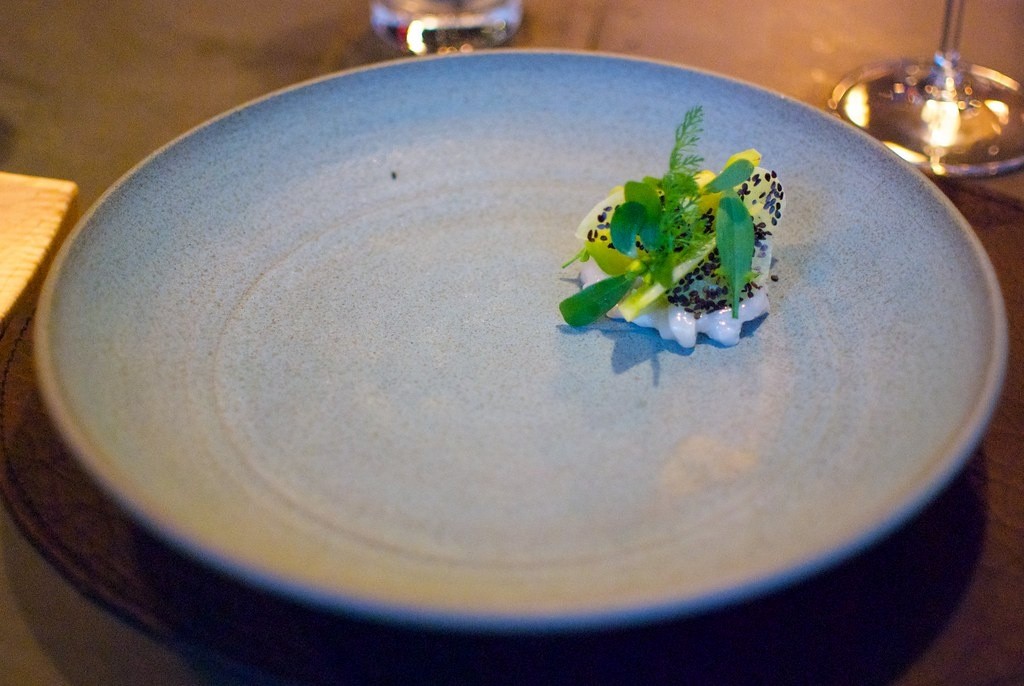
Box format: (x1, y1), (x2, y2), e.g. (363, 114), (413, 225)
(32, 49), (1009, 633)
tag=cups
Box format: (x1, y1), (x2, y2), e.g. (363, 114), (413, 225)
(370, 1), (524, 55)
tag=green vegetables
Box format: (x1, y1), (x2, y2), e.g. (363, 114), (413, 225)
(558, 103), (755, 328)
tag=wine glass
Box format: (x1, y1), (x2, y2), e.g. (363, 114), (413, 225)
(828, 1), (1024, 176)
